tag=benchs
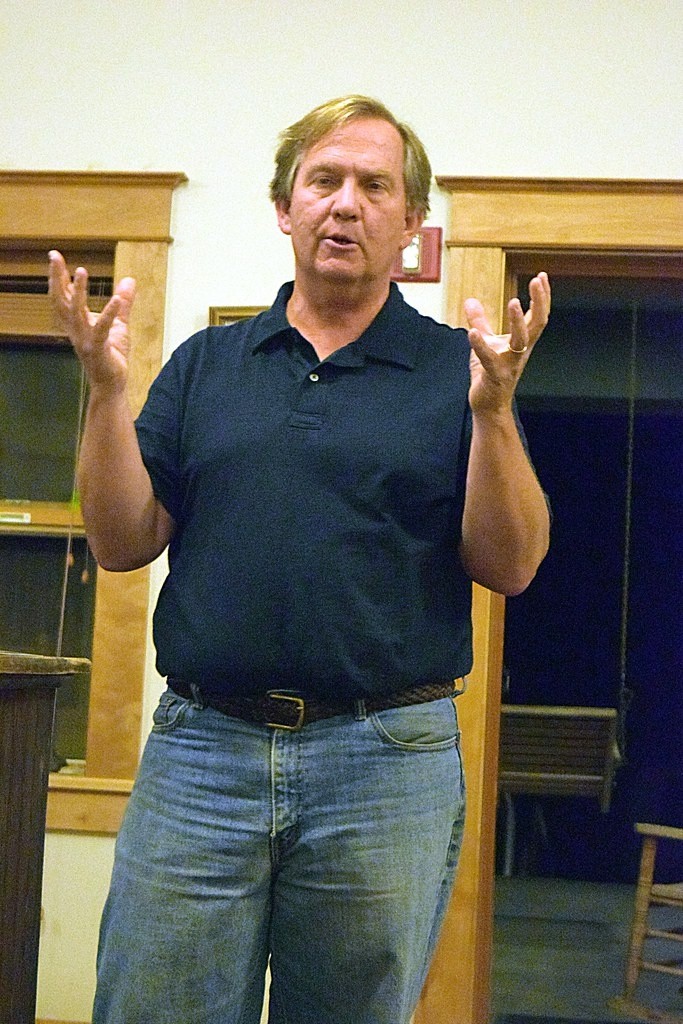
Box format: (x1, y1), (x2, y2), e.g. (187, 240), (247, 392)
(497, 703), (623, 814)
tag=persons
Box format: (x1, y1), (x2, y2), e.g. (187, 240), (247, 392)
(38, 101), (552, 1024)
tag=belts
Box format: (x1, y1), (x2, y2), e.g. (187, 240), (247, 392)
(165, 672), (462, 731)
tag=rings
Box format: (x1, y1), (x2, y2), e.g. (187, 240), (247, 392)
(507, 344), (527, 352)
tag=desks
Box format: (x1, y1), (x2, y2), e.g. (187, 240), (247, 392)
(0, 651), (91, 1024)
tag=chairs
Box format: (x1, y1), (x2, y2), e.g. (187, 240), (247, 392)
(605, 823), (683, 1024)
